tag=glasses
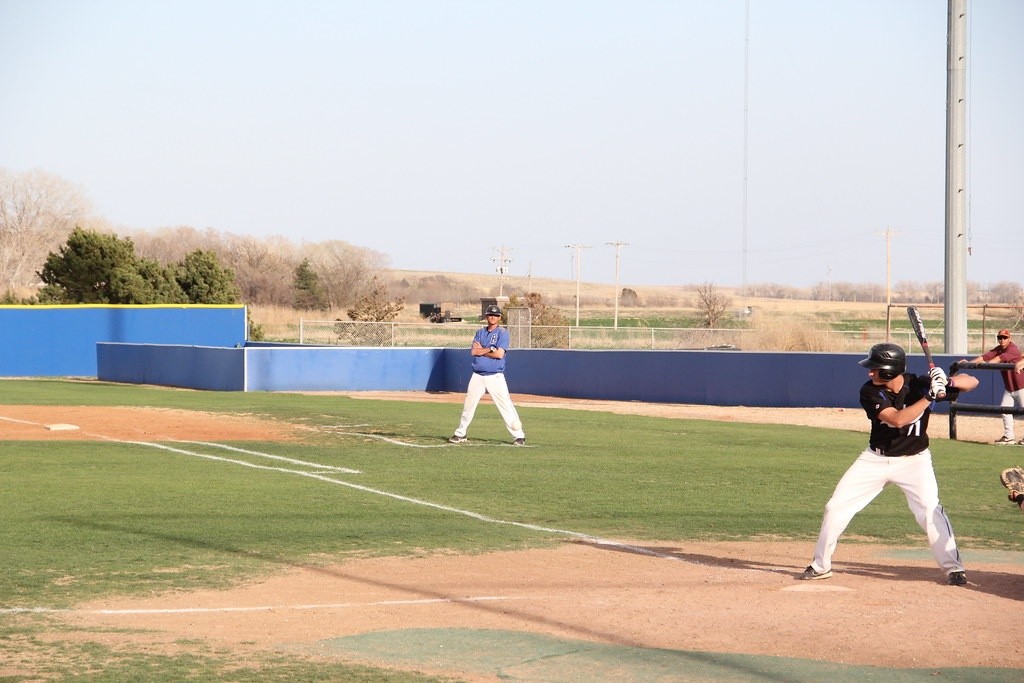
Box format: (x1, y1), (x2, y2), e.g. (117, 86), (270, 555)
(997, 336), (1008, 339)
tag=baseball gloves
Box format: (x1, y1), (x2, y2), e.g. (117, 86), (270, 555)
(999, 465), (1024, 511)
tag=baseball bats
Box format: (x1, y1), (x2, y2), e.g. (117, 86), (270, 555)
(906, 304), (947, 398)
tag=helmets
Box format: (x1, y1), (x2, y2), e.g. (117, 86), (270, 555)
(856, 342), (908, 382)
(485, 306), (503, 316)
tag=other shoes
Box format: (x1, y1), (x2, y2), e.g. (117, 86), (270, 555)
(449, 434), (468, 443)
(513, 437), (525, 447)
(1017, 437), (1024, 446)
(994, 436), (1016, 445)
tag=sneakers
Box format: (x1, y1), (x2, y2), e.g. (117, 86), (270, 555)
(793, 565), (834, 581)
(947, 571), (967, 586)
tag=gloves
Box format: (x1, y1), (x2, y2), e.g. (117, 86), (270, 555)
(926, 367), (952, 390)
(928, 382), (948, 401)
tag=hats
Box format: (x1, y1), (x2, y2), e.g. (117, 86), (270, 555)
(994, 329), (1011, 338)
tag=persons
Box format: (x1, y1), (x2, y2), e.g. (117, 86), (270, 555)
(959, 328), (1024, 510)
(448, 305), (526, 446)
(799, 342), (979, 585)
